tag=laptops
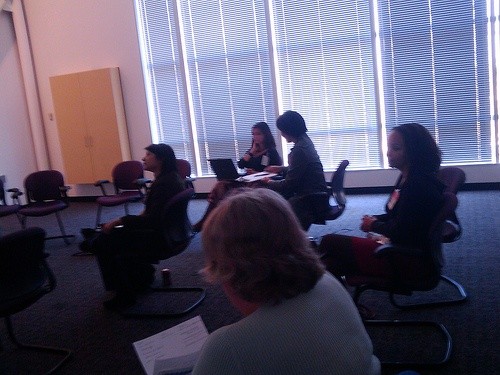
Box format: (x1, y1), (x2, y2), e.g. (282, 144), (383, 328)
(208, 158), (249, 181)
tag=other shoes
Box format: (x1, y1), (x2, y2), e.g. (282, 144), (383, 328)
(103, 295), (136, 309)
(190, 223), (202, 232)
(80, 240), (90, 250)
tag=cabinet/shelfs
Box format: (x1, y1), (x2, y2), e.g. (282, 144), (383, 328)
(48, 68), (131, 186)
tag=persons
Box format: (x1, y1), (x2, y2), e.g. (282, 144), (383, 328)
(192, 189), (382, 375)
(193, 110), (328, 232)
(90, 144), (188, 312)
(318, 122), (443, 298)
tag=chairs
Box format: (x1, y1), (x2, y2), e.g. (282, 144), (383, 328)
(275, 155), (467, 375)
(0, 169), (74, 375)
(93, 160), (205, 320)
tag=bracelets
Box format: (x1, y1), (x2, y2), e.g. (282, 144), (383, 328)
(247, 151), (254, 158)
